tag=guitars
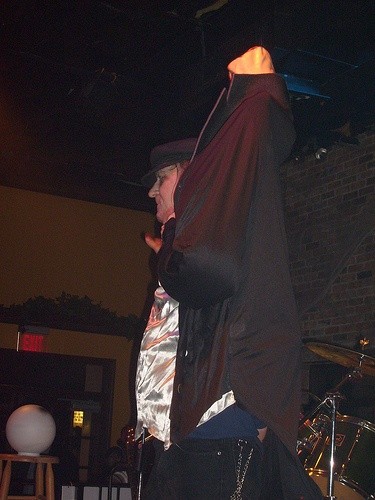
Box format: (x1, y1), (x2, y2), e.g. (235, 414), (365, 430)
(124, 426), (139, 499)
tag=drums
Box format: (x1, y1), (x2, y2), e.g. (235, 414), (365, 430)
(296, 410), (325, 466)
(304, 415), (375, 500)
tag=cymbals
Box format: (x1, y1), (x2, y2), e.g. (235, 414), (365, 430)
(305, 341), (375, 375)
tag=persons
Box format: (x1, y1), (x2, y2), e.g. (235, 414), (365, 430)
(131, 45), (321, 500)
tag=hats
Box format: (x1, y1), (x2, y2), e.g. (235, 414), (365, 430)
(140, 136), (212, 189)
